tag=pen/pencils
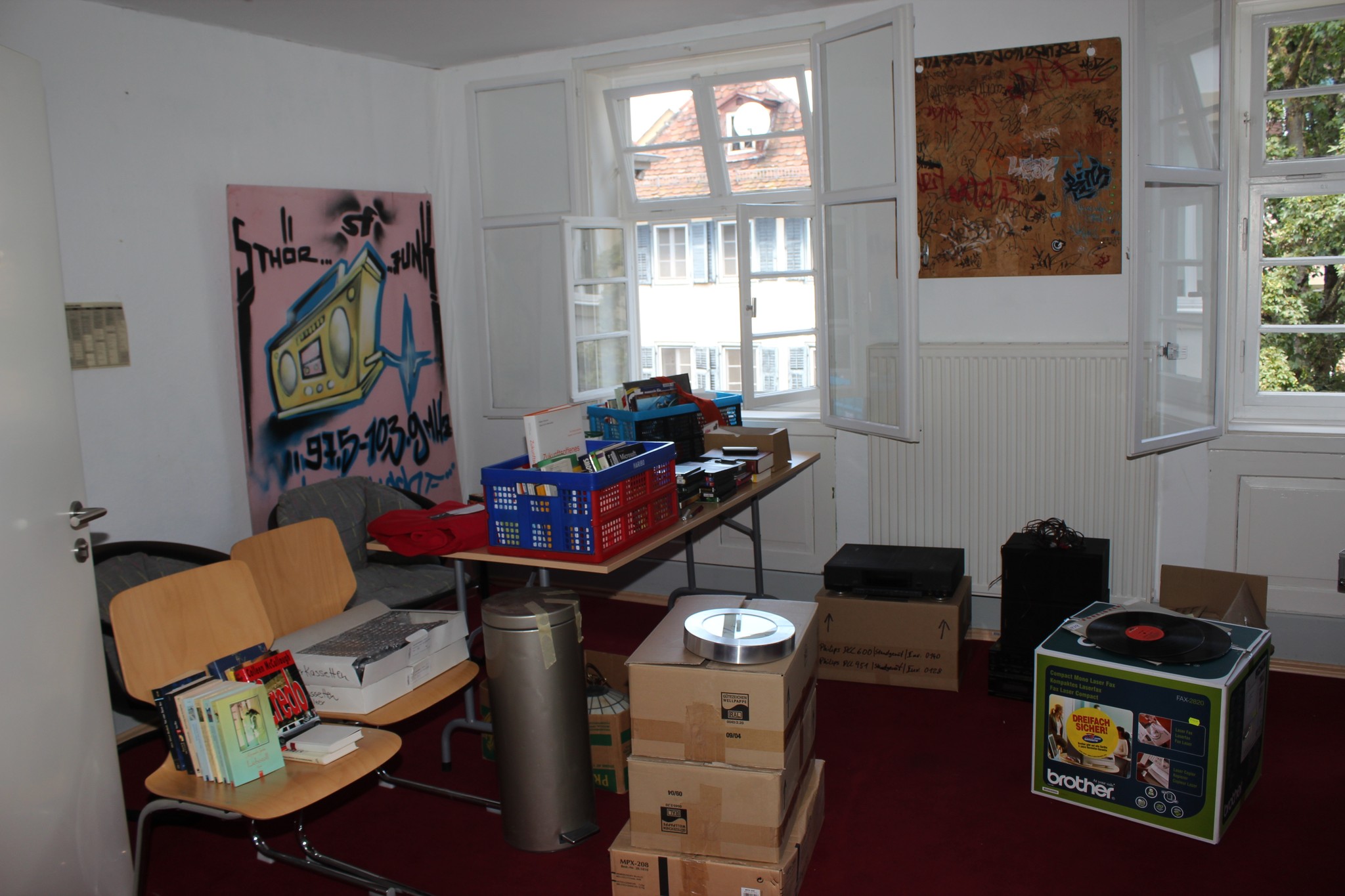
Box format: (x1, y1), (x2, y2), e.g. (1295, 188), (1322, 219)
(689, 505), (704, 519)
(682, 508), (692, 520)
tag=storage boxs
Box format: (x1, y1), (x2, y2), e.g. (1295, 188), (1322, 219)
(608, 759), (827, 896)
(625, 592), (818, 768)
(479, 440), (678, 564)
(815, 575), (975, 694)
(1160, 563), (1268, 629)
(480, 649), (631, 794)
(306, 638), (471, 713)
(704, 425), (793, 472)
(269, 599), (469, 690)
(586, 390), (743, 463)
(1028, 603), (1273, 844)
(625, 689), (817, 862)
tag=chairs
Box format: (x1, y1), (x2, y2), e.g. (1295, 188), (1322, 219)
(229, 518), (506, 884)
(108, 559), (402, 896)
(268, 474), (469, 609)
(91, 541), (230, 728)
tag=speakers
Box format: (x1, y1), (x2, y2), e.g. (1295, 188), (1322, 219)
(1000, 534), (1110, 651)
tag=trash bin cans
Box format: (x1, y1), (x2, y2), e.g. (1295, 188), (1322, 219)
(481, 588), (599, 852)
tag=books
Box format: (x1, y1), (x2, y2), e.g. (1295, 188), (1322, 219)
(699, 448), (774, 483)
(207, 642), (322, 751)
(281, 724), (364, 766)
(605, 373), (679, 438)
(151, 670), (285, 787)
(523, 404), (587, 469)
(492, 441), (646, 550)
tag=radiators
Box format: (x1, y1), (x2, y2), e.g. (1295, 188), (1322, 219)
(865, 341), (1191, 606)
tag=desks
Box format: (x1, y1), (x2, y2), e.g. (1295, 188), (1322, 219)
(363, 449), (820, 769)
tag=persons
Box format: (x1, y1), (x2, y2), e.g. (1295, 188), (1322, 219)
(1113, 726), (1126, 757)
(1048, 705), (1067, 758)
